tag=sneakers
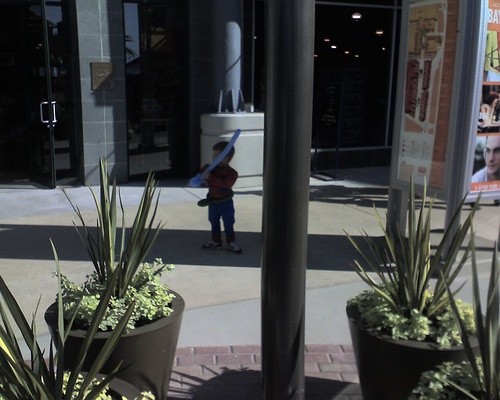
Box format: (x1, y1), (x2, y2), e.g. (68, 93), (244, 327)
(203, 240), (223, 250)
(225, 241), (242, 254)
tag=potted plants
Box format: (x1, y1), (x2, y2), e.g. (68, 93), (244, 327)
(1, 238), (152, 399)
(337, 173), (487, 400)
(43, 156), (185, 400)
(409, 227), (500, 400)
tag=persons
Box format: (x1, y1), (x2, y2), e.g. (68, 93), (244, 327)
(199, 139), (243, 251)
(472, 133), (500, 183)
(479, 90), (499, 132)
